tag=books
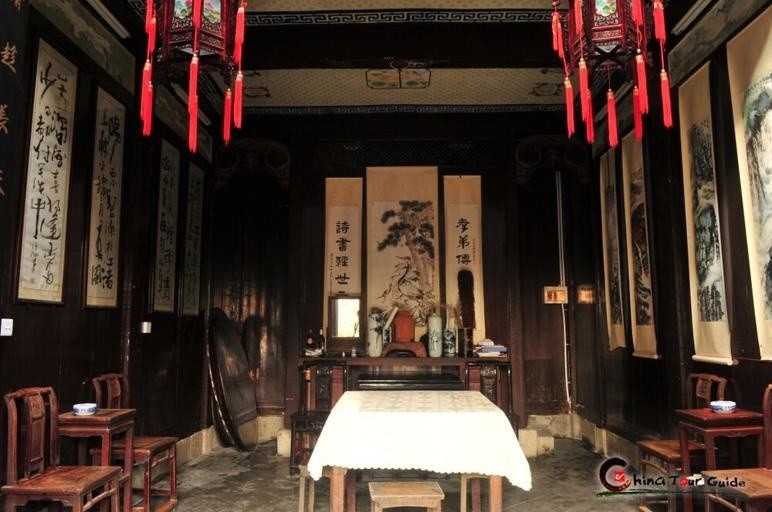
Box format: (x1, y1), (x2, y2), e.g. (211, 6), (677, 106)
(476, 351), (501, 358)
(481, 346), (507, 352)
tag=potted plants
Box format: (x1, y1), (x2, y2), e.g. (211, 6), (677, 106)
(428, 300), (473, 357)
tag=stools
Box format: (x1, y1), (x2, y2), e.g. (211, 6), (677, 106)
(298, 454), (490, 512)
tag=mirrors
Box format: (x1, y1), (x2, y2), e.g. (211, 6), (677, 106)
(326, 294), (364, 352)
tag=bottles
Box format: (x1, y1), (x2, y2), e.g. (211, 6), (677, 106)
(306, 327), (315, 349)
(316, 327), (325, 356)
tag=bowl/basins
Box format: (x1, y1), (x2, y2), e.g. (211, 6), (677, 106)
(709, 399), (737, 415)
(71, 402), (98, 417)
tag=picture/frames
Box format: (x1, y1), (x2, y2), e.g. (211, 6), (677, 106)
(543, 286), (569, 304)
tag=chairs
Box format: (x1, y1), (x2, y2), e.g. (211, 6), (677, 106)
(289, 360), (347, 475)
(635, 373), (728, 512)
(89, 373), (179, 512)
(700, 384), (772, 512)
(465, 361), (519, 438)
(1, 386), (121, 512)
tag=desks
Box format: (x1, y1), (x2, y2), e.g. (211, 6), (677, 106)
(298, 357), (510, 379)
(357, 373), (464, 390)
(306, 391), (532, 512)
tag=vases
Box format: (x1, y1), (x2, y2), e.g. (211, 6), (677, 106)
(367, 313), (456, 357)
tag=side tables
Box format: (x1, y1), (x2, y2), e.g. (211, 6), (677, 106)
(57, 408), (137, 512)
(674, 407), (764, 512)
